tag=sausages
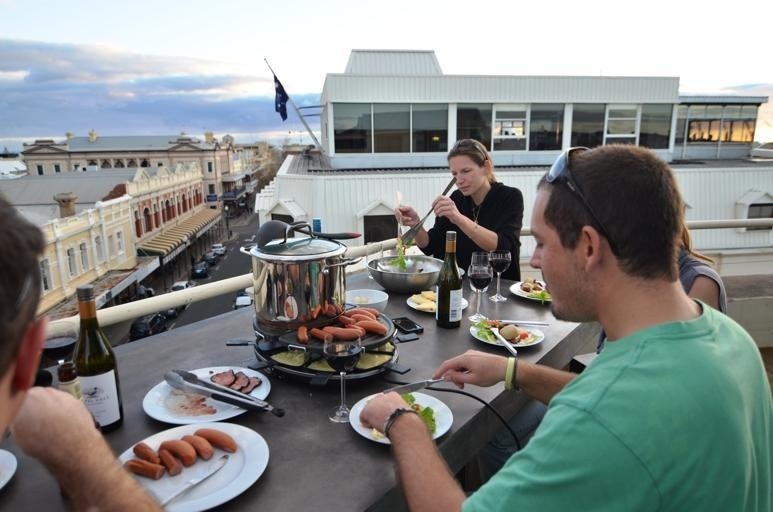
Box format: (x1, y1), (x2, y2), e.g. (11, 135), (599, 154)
(122, 427), (238, 480)
(311, 307), (387, 341)
(298, 326), (308, 343)
(309, 301), (343, 319)
(299, 312), (306, 322)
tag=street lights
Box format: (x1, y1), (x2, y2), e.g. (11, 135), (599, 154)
(224, 205), (230, 239)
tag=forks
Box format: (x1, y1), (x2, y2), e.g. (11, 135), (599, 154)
(489, 326), (518, 356)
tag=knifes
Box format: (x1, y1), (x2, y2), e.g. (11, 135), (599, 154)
(384, 367), (471, 395)
(157, 454), (229, 508)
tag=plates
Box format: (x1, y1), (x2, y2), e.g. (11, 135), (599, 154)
(509, 280), (552, 301)
(119, 422), (270, 512)
(349, 390), (454, 446)
(470, 320), (544, 347)
(0, 449), (17, 492)
(406, 291), (468, 313)
(141, 366), (270, 425)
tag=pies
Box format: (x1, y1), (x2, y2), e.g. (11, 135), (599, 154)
(411, 290), (436, 311)
(269, 350), (305, 368)
(357, 342), (395, 371)
(308, 357), (336, 373)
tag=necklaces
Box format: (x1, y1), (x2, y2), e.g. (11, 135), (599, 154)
(469, 188), (485, 224)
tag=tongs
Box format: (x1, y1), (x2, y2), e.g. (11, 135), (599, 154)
(162, 371), (286, 417)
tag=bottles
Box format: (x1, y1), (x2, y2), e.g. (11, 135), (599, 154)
(56, 285), (124, 512)
(436, 231), (464, 328)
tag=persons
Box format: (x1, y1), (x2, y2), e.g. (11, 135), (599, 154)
(0, 191), (165, 511)
(358, 144), (773, 511)
(395, 140), (525, 280)
(593, 202), (729, 354)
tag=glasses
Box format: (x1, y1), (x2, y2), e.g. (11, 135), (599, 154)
(544, 146), (602, 228)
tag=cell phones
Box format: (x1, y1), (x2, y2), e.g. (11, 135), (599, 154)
(391, 316), (424, 334)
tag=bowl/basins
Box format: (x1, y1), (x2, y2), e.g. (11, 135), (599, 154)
(346, 289), (389, 312)
(366, 255), (444, 293)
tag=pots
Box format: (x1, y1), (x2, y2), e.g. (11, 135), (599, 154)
(240, 221), (363, 329)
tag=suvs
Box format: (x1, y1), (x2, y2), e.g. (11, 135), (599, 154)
(127, 312), (169, 341)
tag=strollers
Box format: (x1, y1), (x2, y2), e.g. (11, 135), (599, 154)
(145, 287), (156, 297)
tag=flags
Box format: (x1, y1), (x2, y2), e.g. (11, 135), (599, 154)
(275, 75), (289, 120)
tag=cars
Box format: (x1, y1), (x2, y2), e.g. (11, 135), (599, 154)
(231, 267), (255, 310)
(157, 303), (187, 319)
(191, 243), (227, 279)
(167, 280), (198, 294)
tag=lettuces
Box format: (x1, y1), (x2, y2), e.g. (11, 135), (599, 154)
(529, 290), (552, 304)
(396, 394), (436, 436)
(477, 318), (494, 341)
(389, 241), (407, 272)
(288, 280), (294, 294)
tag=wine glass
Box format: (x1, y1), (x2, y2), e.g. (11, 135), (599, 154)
(467, 251), (511, 323)
(323, 332), (362, 423)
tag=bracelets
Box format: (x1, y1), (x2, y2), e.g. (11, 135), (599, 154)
(504, 354), (519, 396)
(384, 407), (420, 439)
(467, 220), (480, 239)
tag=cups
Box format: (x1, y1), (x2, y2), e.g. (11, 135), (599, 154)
(42, 320), (78, 365)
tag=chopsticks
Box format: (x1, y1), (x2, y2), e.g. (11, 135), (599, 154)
(395, 191), (404, 245)
